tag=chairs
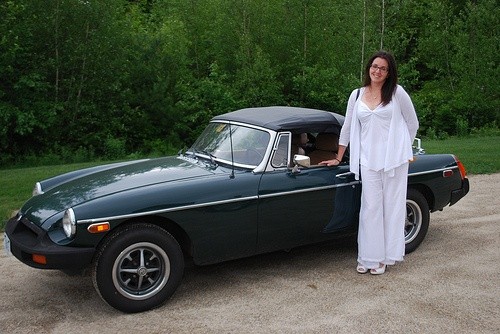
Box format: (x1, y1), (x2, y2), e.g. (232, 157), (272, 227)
(273, 132), (308, 167)
(309, 132), (339, 165)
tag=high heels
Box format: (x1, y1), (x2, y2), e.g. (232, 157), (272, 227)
(356, 263), (368, 273)
(369, 264), (387, 275)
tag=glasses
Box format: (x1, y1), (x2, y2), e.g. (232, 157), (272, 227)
(370, 64), (389, 73)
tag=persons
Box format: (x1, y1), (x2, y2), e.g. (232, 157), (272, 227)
(318, 50), (419, 275)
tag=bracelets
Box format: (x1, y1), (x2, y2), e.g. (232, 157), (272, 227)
(334, 157), (342, 163)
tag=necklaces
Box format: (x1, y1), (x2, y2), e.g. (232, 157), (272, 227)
(367, 86), (382, 99)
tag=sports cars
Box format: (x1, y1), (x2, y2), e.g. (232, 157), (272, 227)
(3, 106), (470, 313)
